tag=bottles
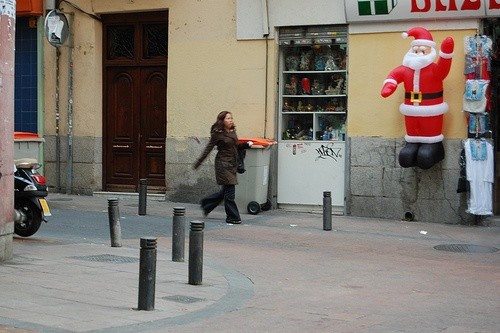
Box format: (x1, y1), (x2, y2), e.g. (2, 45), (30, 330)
(285, 53), (301, 70)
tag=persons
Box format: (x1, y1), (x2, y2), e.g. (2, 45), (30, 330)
(382, 26), (454, 169)
(194, 111), (253, 224)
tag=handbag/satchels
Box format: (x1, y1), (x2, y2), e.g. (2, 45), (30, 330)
(237, 149), (246, 174)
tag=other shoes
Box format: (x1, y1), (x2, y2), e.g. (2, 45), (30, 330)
(200, 205), (209, 218)
(225, 219), (241, 224)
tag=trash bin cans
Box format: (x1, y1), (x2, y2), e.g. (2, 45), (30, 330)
(234, 137), (279, 213)
(14, 131), (45, 178)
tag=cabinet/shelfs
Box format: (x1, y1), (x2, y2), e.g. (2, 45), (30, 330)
(278, 48), (347, 141)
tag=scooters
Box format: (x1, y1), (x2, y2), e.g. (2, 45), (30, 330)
(13, 157), (52, 238)
